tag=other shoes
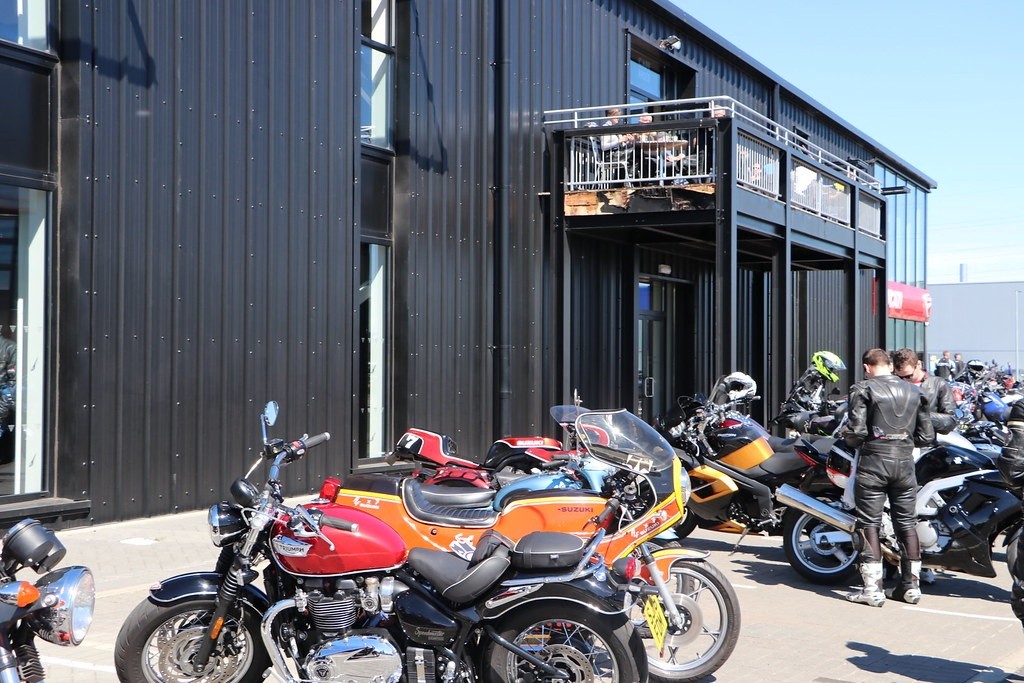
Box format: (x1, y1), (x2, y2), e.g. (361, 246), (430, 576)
(920, 568), (936, 584)
(886, 565), (899, 580)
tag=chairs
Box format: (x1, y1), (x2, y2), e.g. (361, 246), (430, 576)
(678, 146), (706, 184)
(586, 120), (634, 188)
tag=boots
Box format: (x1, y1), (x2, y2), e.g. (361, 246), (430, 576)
(846, 561), (886, 607)
(884, 560), (921, 603)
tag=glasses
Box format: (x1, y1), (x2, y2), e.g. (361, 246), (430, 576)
(898, 366), (917, 379)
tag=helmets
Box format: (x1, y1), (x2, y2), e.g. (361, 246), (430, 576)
(967, 360), (986, 374)
(826, 439), (859, 489)
(810, 350), (847, 383)
(723, 372), (757, 401)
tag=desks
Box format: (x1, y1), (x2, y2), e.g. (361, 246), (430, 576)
(636, 138), (689, 188)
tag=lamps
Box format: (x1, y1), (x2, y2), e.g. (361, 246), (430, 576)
(657, 262), (672, 274)
(660, 34), (683, 52)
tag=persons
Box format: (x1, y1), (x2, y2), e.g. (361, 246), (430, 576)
(843, 347), (935, 607)
(934, 351), (956, 381)
(737, 147), (854, 219)
(892, 348), (959, 435)
(953, 353), (967, 381)
(601, 105), (726, 188)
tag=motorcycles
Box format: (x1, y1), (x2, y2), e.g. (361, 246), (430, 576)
(0, 518), (95, 683)
(114, 400), (667, 683)
(384, 388), (695, 638)
(647, 359), (1024, 629)
(319, 408), (741, 683)
